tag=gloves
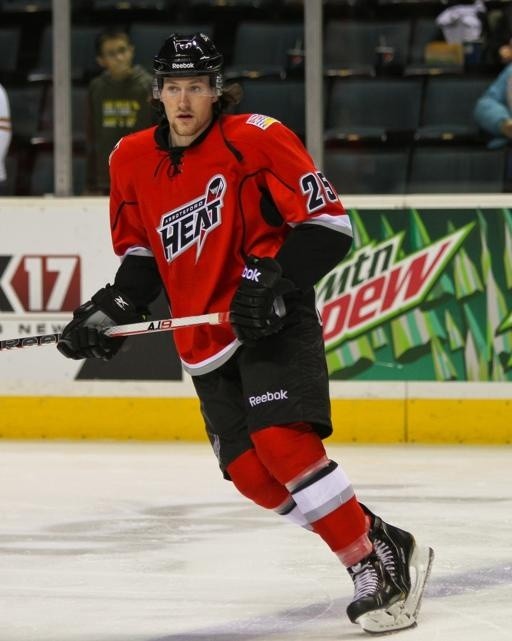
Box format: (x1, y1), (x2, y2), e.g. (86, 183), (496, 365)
(231, 254), (297, 343)
(57, 282), (152, 362)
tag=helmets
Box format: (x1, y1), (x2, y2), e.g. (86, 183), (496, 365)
(148, 30), (225, 98)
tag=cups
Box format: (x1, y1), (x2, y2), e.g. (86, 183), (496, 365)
(288, 49), (304, 79)
(374, 47), (397, 62)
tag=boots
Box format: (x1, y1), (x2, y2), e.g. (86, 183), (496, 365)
(346, 550), (405, 619)
(353, 500), (414, 597)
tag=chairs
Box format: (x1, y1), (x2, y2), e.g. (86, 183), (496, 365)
(40, 151), (96, 198)
(219, 80), (308, 142)
(412, 20), (488, 75)
(41, 81), (91, 149)
(327, 77), (422, 146)
(412, 147), (506, 196)
(322, 147), (410, 196)
(124, 18), (215, 76)
(40, 21), (108, 84)
(229, 19), (307, 78)
(321, 20), (411, 75)
(423, 76), (495, 143)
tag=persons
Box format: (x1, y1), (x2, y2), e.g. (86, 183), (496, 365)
(57, 32), (417, 627)
(81, 29), (160, 196)
(472, 56), (511, 137)
